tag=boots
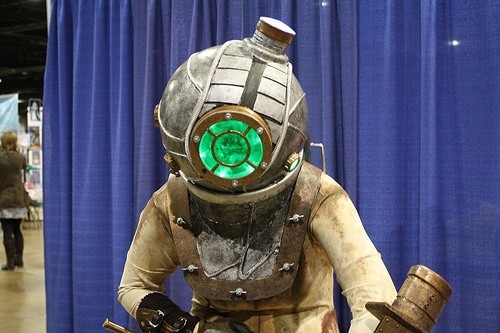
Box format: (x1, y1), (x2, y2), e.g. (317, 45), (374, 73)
(2, 237), (23, 271)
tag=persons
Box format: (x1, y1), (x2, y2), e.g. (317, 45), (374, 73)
(117, 17), (397, 333)
(0, 131), (30, 270)
